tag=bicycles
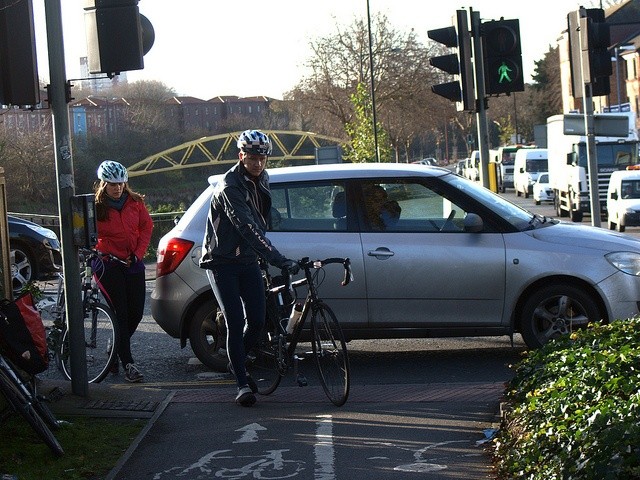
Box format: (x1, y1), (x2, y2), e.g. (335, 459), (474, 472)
(58, 247), (127, 385)
(1, 310), (64, 455)
(214, 258), (351, 405)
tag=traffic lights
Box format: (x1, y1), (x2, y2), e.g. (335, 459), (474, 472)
(0, 0), (40, 106)
(484, 20), (525, 95)
(428, 11), (475, 113)
(580, 7), (611, 97)
(90, 0), (155, 74)
(567, 12), (581, 98)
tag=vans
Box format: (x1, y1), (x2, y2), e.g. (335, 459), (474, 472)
(514, 149), (548, 197)
(465, 158), (471, 178)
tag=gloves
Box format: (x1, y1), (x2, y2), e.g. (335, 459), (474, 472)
(280, 256), (299, 274)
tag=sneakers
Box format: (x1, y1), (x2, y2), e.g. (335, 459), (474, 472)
(124, 363), (143, 383)
(228, 360), (258, 392)
(109, 350), (119, 373)
(237, 384), (253, 405)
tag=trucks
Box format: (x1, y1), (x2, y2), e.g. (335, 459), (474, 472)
(496, 146), (539, 192)
(471, 151), (479, 181)
(547, 112), (639, 222)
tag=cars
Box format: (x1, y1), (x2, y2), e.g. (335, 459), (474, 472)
(532, 172), (555, 205)
(454, 160), (465, 175)
(150, 163), (638, 372)
(607, 166), (640, 232)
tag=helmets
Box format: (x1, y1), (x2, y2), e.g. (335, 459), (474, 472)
(97, 160), (128, 183)
(237, 129), (272, 154)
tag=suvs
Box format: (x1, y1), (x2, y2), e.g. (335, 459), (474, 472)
(8, 215), (61, 297)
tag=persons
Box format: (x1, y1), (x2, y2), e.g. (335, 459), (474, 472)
(199, 129), (301, 408)
(366, 186), (400, 232)
(92, 158), (155, 382)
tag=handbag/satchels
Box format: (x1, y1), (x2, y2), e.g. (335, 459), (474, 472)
(1, 290), (49, 373)
